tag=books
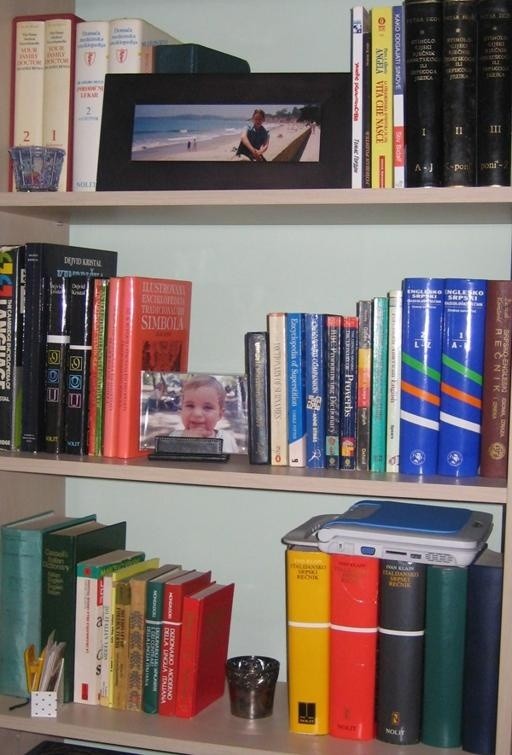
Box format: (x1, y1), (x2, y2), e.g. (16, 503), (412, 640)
(244, 277), (510, 480)
(285, 546), (502, 755)
(0, 512), (236, 720)
(352, 0), (511, 187)
(0, 241), (191, 460)
(11, 15), (249, 193)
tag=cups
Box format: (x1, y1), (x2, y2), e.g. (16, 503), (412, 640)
(225, 655), (280, 720)
(8, 146), (65, 192)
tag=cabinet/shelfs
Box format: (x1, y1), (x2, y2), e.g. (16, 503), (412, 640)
(0, 187), (512, 755)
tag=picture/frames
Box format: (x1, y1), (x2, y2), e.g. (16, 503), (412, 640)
(97, 70), (351, 189)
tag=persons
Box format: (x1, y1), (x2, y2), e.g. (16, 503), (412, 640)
(310, 121), (316, 133)
(236, 108), (270, 162)
(180, 375), (239, 453)
